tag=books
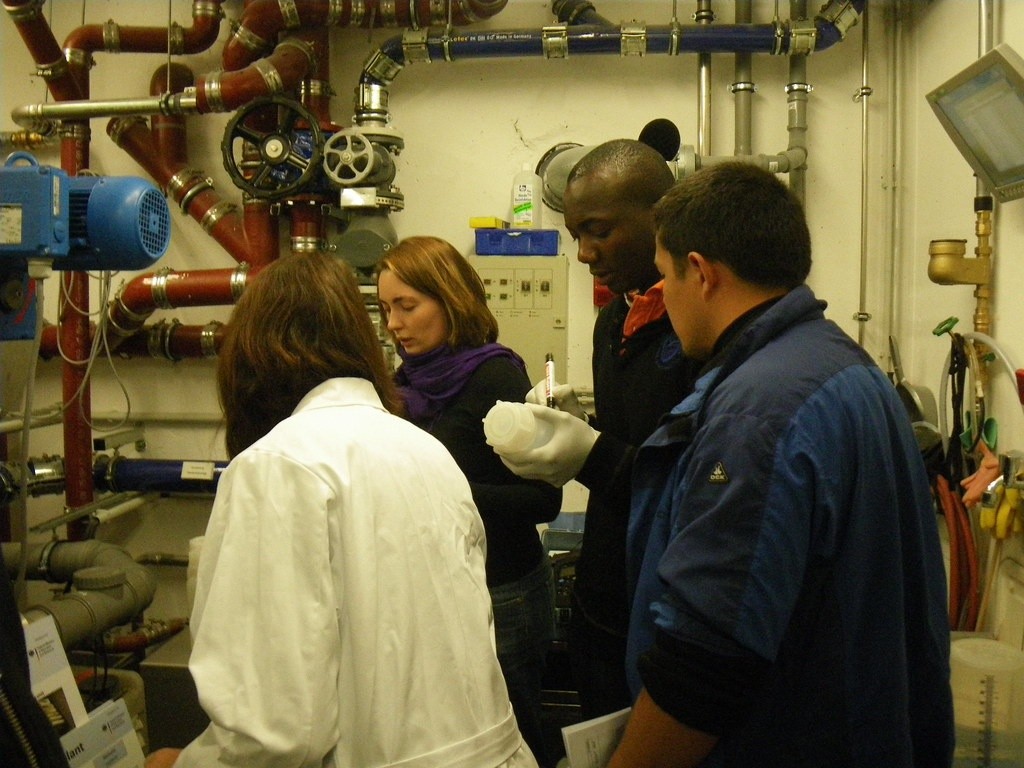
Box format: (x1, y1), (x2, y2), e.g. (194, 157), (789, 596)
(562, 707), (631, 768)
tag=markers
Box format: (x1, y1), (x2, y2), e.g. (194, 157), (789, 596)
(545, 352), (557, 410)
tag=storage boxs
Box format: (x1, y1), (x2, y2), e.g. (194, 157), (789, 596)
(469, 217), (512, 230)
(475, 228), (562, 256)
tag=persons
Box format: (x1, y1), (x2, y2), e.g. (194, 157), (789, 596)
(497, 139), (708, 721)
(375, 236), (567, 768)
(604, 162), (957, 768)
(137, 252), (540, 768)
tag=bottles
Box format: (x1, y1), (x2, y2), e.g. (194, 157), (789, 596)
(483, 399), (555, 454)
(511, 161), (543, 231)
